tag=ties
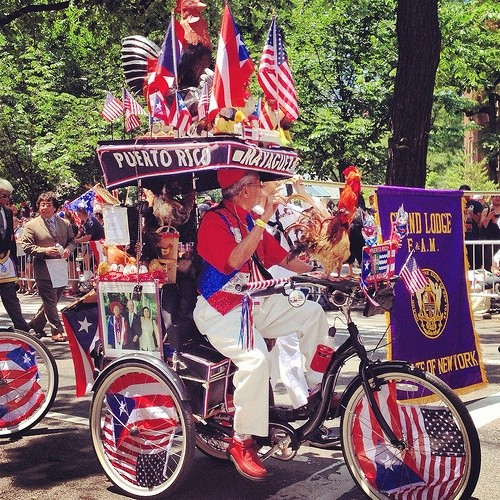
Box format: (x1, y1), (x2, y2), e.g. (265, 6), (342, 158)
(0, 207), (5, 240)
(130, 313), (132, 325)
(48, 220), (56, 237)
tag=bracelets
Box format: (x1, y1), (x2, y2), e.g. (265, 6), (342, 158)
(256, 219), (267, 229)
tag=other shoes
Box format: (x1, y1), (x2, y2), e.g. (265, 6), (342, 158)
(52, 334), (66, 342)
(301, 425), (341, 447)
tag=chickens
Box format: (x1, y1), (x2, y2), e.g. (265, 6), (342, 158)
(97, 238), (137, 267)
(138, 185), (199, 235)
(282, 164), (379, 280)
(84, 182), (140, 257)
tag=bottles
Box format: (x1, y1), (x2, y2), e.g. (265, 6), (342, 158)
(318, 325), (336, 358)
(56, 243), (67, 261)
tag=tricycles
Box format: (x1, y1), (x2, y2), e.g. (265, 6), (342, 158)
(60, 133), (481, 500)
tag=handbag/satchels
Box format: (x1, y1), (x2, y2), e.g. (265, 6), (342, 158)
(151, 319), (158, 348)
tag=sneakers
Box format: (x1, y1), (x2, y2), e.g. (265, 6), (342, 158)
(225, 436), (268, 482)
(308, 383), (341, 413)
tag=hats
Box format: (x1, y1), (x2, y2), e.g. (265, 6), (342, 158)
(217, 169), (248, 189)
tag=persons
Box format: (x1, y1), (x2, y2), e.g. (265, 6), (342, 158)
(0, 178), (106, 344)
(192, 169), (345, 482)
(251, 176), (341, 447)
(347, 192), (366, 279)
(134, 182), (199, 320)
(459, 184), (500, 276)
(106, 300), (160, 350)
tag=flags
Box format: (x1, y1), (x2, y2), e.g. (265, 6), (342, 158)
(399, 254), (429, 295)
(101, 5), (298, 147)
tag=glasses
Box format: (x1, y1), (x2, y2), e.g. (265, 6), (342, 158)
(0, 193), (11, 199)
(247, 181), (264, 187)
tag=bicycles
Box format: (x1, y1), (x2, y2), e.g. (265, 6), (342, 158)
(0, 325), (58, 439)
